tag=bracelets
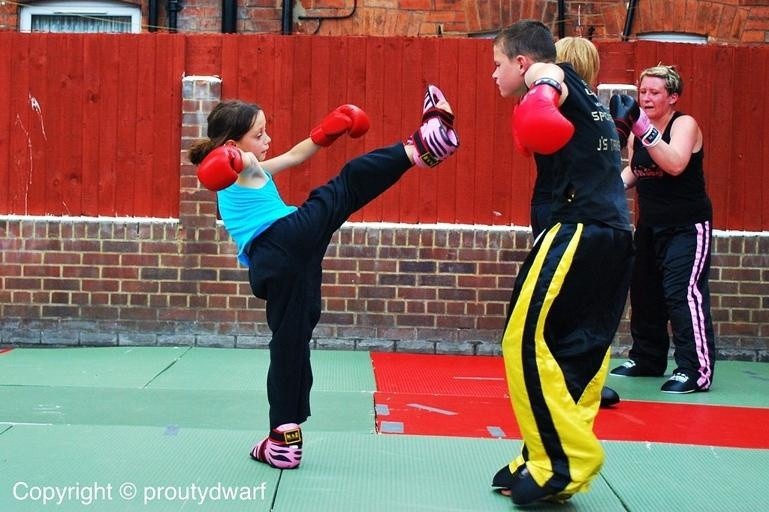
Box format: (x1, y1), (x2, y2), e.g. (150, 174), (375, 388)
(639, 124), (662, 147)
(530, 77), (562, 96)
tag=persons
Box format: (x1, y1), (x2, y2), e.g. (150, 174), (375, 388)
(189, 84), (460, 470)
(492, 17), (633, 507)
(553, 36), (638, 405)
(610, 64), (713, 394)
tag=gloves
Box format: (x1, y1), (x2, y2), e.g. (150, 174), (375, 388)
(610, 93), (663, 152)
(511, 78), (577, 158)
(196, 146), (244, 192)
(310, 103), (371, 148)
